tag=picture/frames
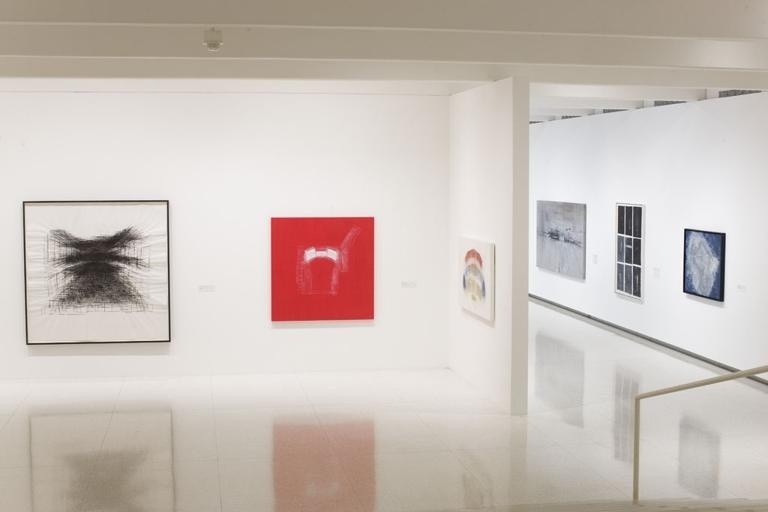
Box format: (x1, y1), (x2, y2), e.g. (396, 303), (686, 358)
(683, 229), (725, 301)
(23, 200), (171, 345)
